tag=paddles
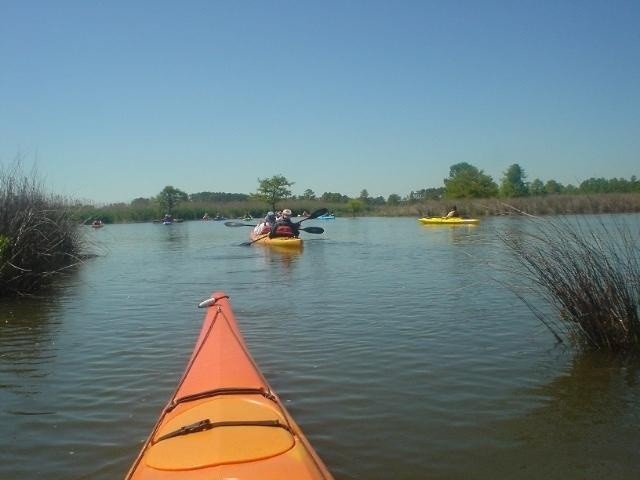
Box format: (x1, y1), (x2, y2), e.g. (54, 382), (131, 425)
(224, 222), (256, 228)
(299, 227), (324, 234)
(239, 235), (269, 246)
(297, 208), (326, 223)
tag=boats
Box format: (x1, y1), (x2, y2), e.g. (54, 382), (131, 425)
(249, 228), (305, 250)
(416, 214), (482, 225)
(161, 212), (337, 225)
(124, 290), (337, 479)
(91, 223), (106, 228)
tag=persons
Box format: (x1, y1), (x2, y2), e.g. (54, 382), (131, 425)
(265, 209), (283, 226)
(268, 209), (302, 239)
(92, 218), (102, 225)
(303, 210), (311, 218)
(163, 213), (174, 222)
(202, 212), (209, 221)
(244, 211), (253, 220)
(446, 205), (459, 218)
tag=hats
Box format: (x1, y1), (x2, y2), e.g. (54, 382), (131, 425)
(265, 211), (276, 223)
(283, 209), (292, 216)
